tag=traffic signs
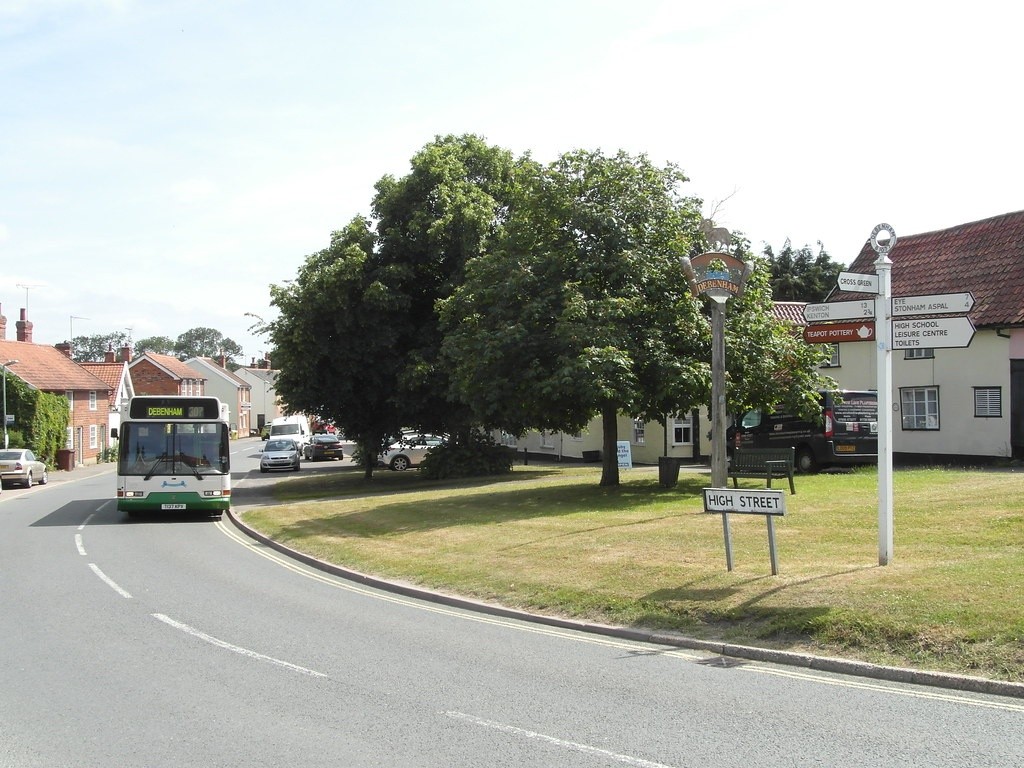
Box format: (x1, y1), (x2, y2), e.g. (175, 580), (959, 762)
(802, 272), (977, 349)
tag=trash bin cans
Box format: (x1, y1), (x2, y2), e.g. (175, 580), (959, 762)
(55, 449), (75, 472)
(658, 456), (682, 488)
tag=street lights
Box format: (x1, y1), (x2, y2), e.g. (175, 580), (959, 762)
(263, 381), (270, 425)
(3, 360), (19, 448)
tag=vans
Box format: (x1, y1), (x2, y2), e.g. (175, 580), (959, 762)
(269, 414), (309, 455)
(727, 389), (877, 474)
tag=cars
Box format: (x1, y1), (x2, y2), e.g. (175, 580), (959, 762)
(259, 438), (301, 471)
(0, 448), (48, 488)
(312, 422), (337, 435)
(261, 424), (272, 441)
(377, 430), (459, 471)
(302, 435), (344, 461)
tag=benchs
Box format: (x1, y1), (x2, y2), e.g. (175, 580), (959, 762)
(727, 447), (797, 494)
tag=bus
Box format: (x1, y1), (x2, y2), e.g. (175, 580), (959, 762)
(111, 395), (239, 517)
